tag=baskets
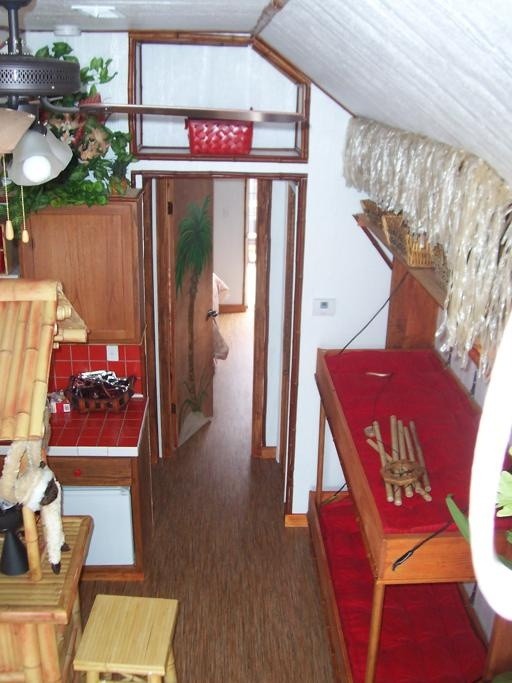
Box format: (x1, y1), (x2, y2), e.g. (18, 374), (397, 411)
(185, 116), (253, 155)
(64, 375), (133, 415)
(382, 214), (433, 268)
(432, 243), (452, 294)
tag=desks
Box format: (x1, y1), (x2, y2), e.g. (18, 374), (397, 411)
(2, 501), (95, 683)
(310, 352), (496, 680)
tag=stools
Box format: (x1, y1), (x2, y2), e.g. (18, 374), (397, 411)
(75, 595), (187, 683)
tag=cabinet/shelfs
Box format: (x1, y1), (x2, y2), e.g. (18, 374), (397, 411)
(44, 456), (139, 577)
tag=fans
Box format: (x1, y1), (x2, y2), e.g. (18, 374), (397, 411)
(1, 1), (313, 150)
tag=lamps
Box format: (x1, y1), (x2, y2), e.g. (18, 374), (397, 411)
(8, 116), (73, 194)
